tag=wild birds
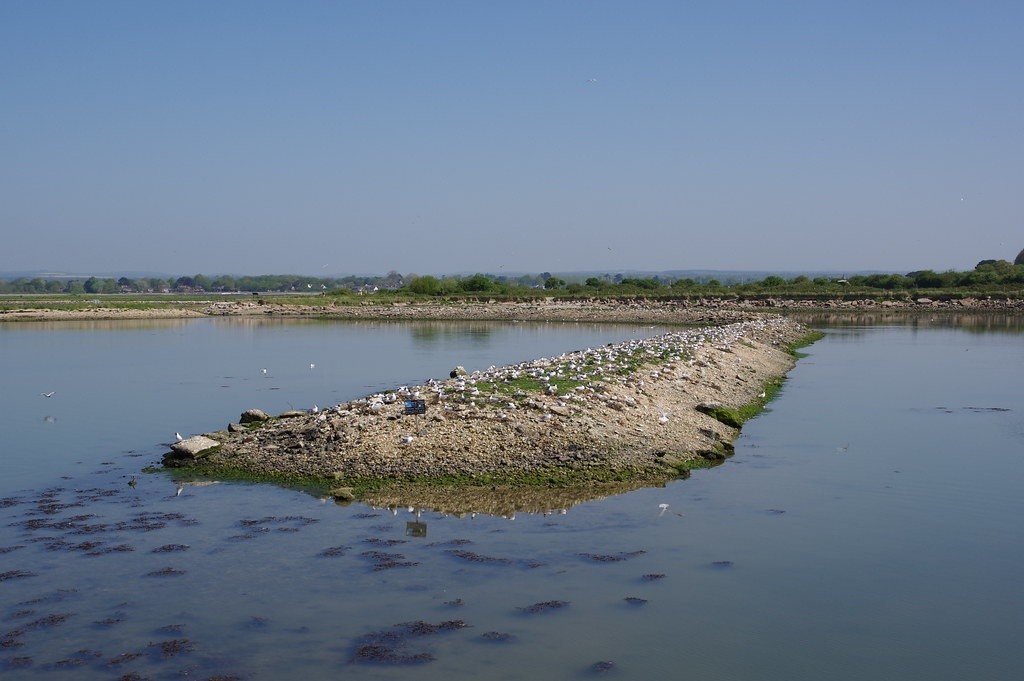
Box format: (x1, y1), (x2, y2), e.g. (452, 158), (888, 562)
(174, 318), (803, 444)
(42, 391), (55, 397)
